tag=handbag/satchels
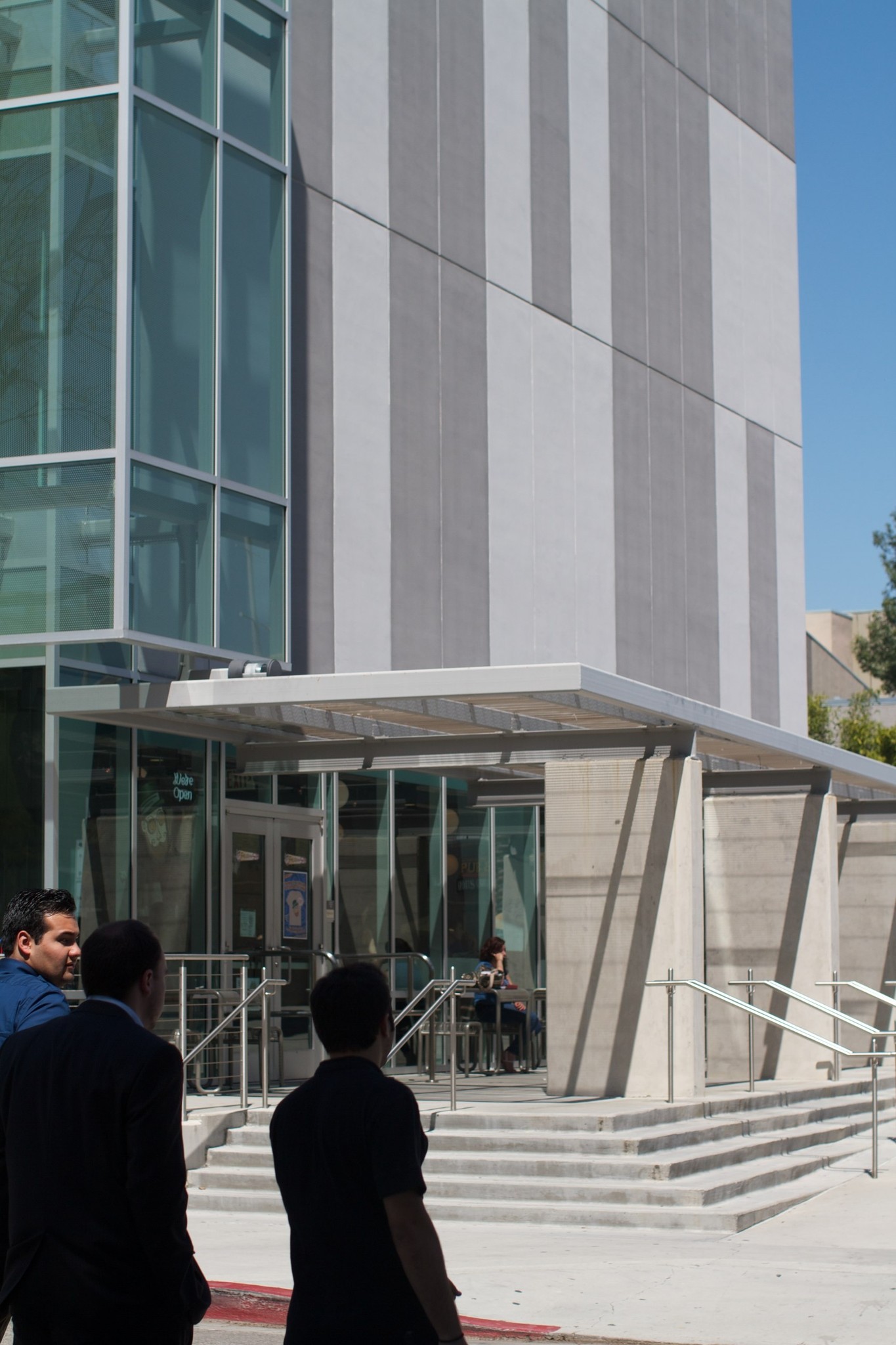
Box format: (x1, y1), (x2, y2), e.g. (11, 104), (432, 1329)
(476, 965), (504, 990)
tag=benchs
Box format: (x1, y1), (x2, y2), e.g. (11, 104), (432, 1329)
(456, 981), (546, 1075)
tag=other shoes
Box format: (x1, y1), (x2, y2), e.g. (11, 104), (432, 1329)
(500, 1051), (516, 1073)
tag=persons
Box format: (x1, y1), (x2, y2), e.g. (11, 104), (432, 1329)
(473, 937), (541, 1074)
(0, 888), (211, 1345)
(269, 960), (470, 1345)
(381, 938), (425, 1067)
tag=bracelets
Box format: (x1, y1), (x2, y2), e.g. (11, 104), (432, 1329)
(439, 1332), (464, 1342)
